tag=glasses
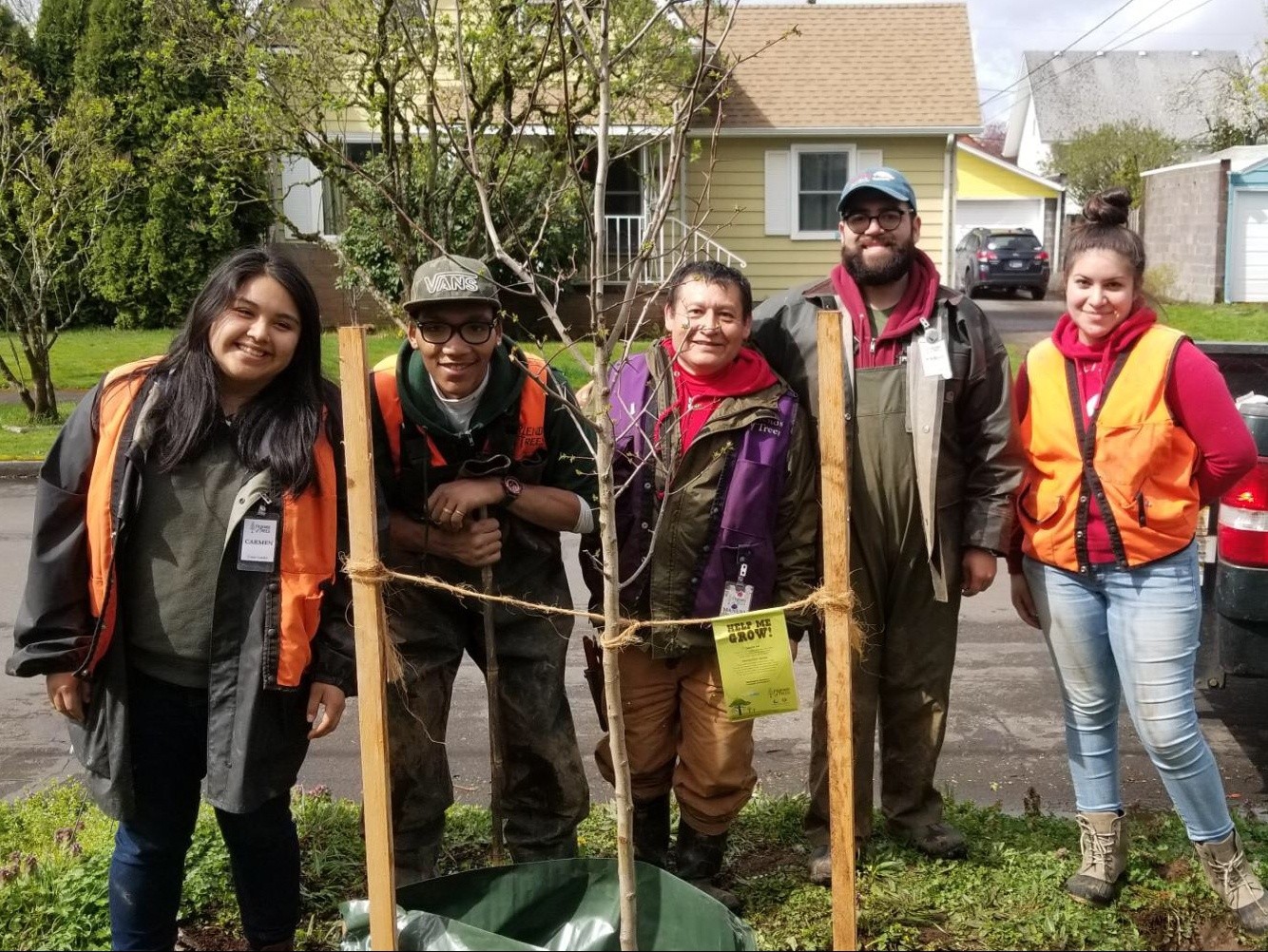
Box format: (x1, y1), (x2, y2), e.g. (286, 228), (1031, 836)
(412, 315), (498, 345)
(843, 209), (913, 235)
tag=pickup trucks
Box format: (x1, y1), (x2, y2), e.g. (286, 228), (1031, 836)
(1187, 334), (1268, 796)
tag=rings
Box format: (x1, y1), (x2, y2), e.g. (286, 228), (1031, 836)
(456, 509), (465, 517)
(443, 508), (453, 514)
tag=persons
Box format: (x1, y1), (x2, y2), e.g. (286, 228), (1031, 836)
(574, 261), (819, 915)
(750, 166), (1026, 886)
(357, 253), (601, 888)
(6, 248), (359, 952)
(1004, 188), (1268, 934)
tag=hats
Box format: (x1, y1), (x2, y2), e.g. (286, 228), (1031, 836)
(836, 166), (918, 216)
(402, 255), (501, 318)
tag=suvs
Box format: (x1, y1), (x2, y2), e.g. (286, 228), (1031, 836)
(955, 226), (1053, 300)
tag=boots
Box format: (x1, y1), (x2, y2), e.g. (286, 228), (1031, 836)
(1193, 828), (1268, 934)
(674, 818), (741, 911)
(632, 795), (670, 870)
(1067, 809), (1129, 907)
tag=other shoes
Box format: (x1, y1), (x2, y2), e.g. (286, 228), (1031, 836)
(809, 842), (860, 885)
(886, 820), (968, 857)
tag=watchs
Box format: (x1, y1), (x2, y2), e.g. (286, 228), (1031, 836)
(978, 547), (999, 557)
(500, 475), (524, 509)
